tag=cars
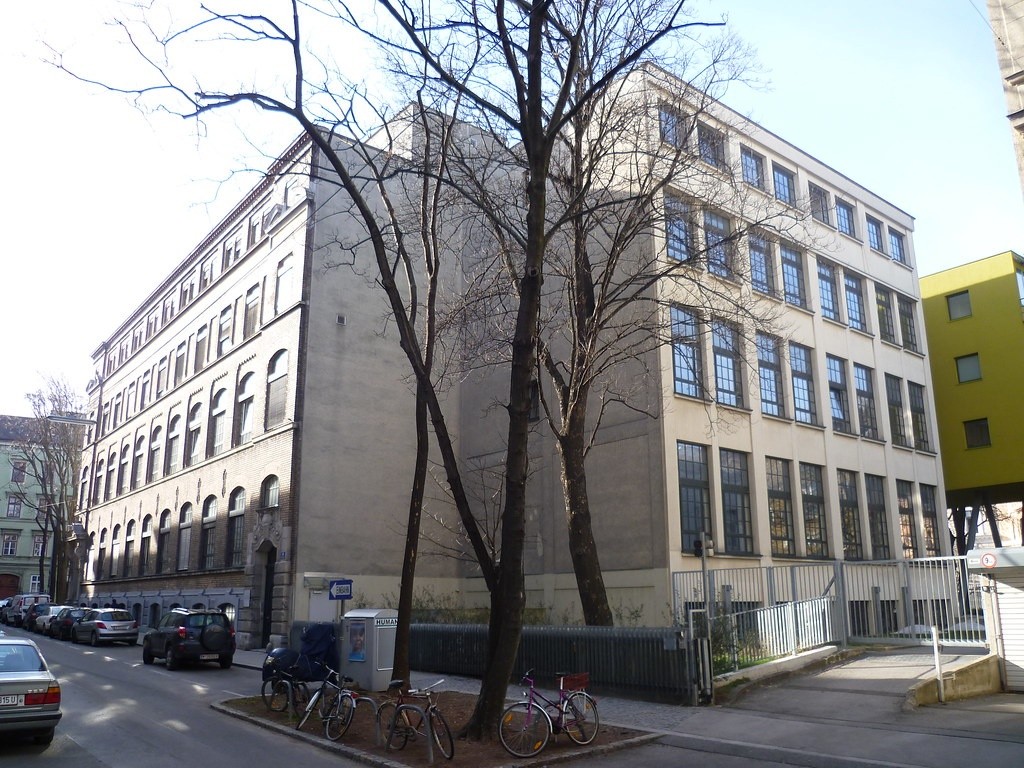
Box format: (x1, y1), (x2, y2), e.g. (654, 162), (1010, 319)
(48, 607), (93, 641)
(0, 634), (63, 745)
(0, 594), (74, 635)
(71, 608), (139, 647)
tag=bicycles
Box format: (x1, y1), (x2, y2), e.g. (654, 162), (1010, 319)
(375, 678), (455, 760)
(295, 664), (359, 741)
(497, 668), (600, 759)
(261, 653), (311, 719)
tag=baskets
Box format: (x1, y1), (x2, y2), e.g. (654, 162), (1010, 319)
(555, 673), (590, 691)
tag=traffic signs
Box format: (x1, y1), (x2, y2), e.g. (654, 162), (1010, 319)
(328, 579), (353, 601)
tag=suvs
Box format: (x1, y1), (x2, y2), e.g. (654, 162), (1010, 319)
(143, 607), (237, 671)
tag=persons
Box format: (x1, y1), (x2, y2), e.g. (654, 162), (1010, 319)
(348, 623), (364, 659)
(111, 599), (119, 608)
